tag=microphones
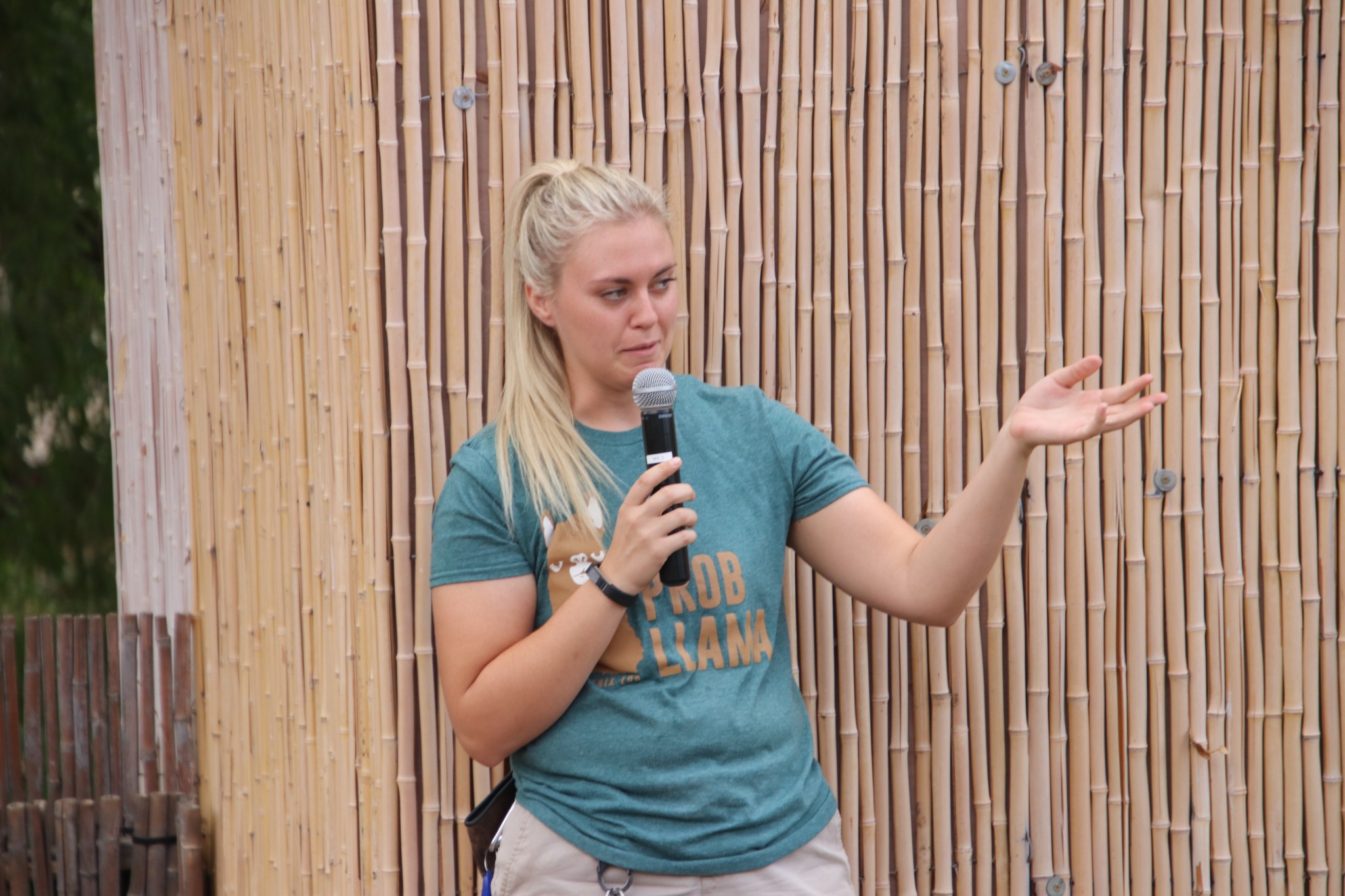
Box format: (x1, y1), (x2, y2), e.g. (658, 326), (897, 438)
(633, 369), (695, 586)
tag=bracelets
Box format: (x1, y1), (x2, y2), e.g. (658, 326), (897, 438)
(585, 561), (639, 607)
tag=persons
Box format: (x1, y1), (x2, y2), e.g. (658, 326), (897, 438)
(426, 157), (1171, 896)
